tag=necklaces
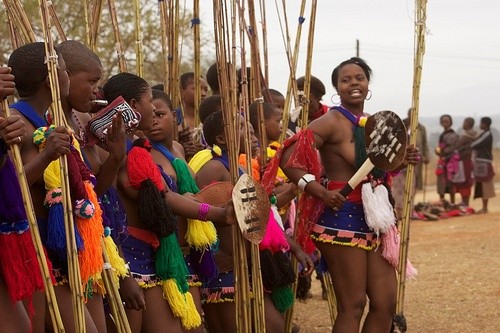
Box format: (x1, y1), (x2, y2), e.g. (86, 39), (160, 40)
(70, 111), (87, 150)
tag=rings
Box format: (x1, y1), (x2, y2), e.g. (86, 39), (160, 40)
(17, 136), (21, 142)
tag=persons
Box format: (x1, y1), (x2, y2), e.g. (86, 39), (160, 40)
(0, 39), (429, 333)
(433, 113), (497, 214)
